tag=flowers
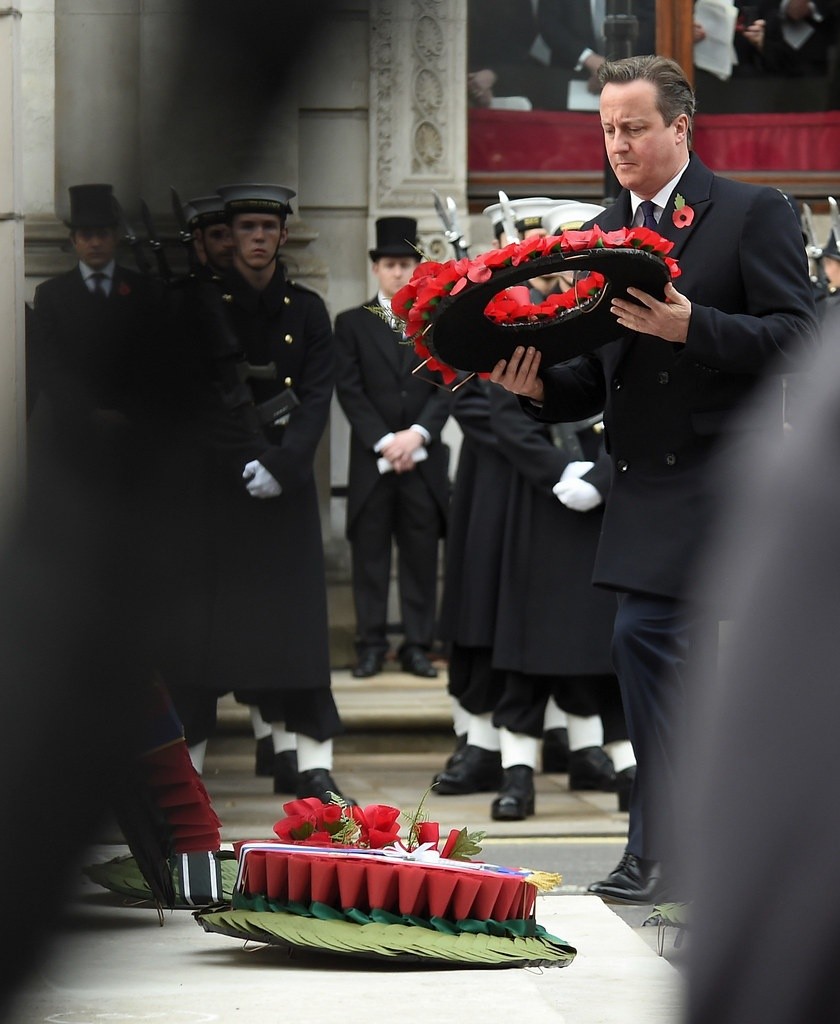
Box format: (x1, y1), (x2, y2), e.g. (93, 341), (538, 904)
(118, 280), (129, 293)
(672, 194), (695, 230)
(273, 782), (486, 862)
(363, 221), (682, 385)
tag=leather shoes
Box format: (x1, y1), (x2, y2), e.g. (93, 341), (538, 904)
(255, 734), (358, 806)
(353, 644), (392, 677)
(398, 644), (438, 678)
(587, 851), (679, 905)
(431, 727), (638, 820)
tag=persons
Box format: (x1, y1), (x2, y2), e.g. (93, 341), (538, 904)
(467, 0), (840, 112)
(331, 215), (474, 680)
(488, 55), (822, 893)
(430, 197), (840, 1024)
(0, 183), (355, 909)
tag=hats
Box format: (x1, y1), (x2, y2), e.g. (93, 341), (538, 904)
(216, 183), (297, 216)
(179, 203), (202, 232)
(482, 197), (553, 238)
(190, 196), (226, 227)
(369, 216), (426, 262)
(541, 203), (607, 236)
(509, 200), (581, 233)
(62, 183), (119, 230)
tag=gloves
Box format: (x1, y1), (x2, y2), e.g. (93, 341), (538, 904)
(242, 459), (283, 499)
(552, 477), (602, 512)
(559, 460), (595, 482)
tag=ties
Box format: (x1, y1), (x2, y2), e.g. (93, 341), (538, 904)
(640, 201), (658, 231)
(88, 272), (111, 297)
